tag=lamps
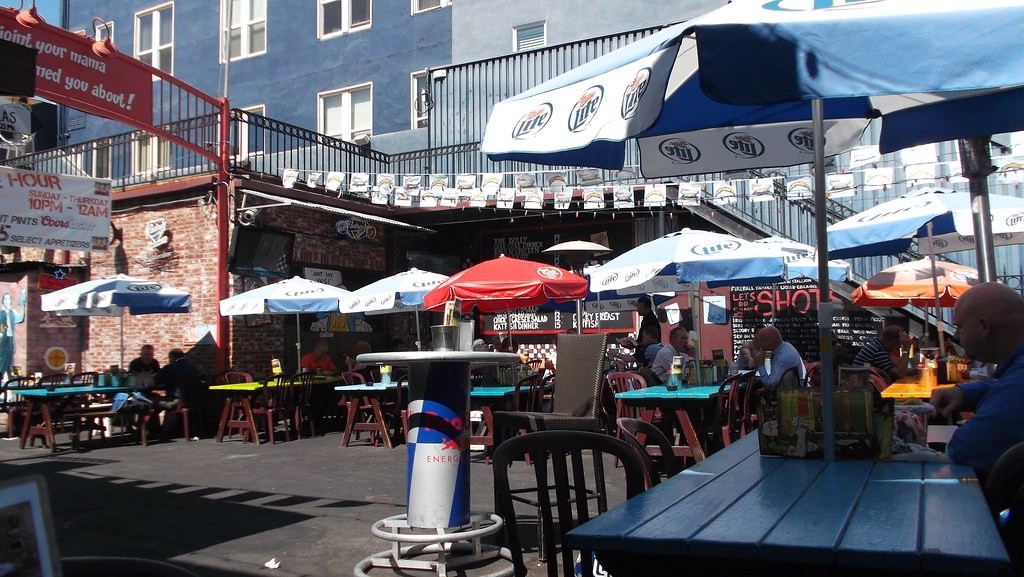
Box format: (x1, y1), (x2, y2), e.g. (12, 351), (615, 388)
(541, 241), (614, 334)
(353, 134), (370, 145)
(8, 0), (47, 30)
(82, 17), (118, 59)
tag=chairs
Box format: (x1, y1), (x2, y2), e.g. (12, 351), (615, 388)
(496, 430), (645, 577)
(0, 360), (825, 491)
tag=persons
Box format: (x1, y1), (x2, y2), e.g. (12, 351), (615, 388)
(620, 297), (661, 350)
(301, 340), (336, 375)
(345, 340), (381, 384)
(852, 324), (918, 384)
(736, 324), (766, 370)
(651, 326), (695, 383)
(961, 359), (987, 379)
(0, 293), (25, 380)
(929, 281), (1024, 479)
(738, 325), (806, 415)
(128, 344), (198, 408)
(607, 326), (664, 369)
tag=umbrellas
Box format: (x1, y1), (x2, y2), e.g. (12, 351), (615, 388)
(337, 267), (450, 351)
(827, 187), (1024, 356)
(481, 0), (1024, 462)
(41, 273), (193, 369)
(706, 235), (850, 326)
(849, 255), (1003, 348)
(220, 276), (351, 381)
(589, 227), (785, 387)
(423, 253), (588, 382)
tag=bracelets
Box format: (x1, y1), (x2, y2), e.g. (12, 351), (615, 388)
(902, 351), (908, 354)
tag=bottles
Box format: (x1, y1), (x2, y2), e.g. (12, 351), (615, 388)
(906, 344), (918, 376)
(950, 358), (959, 382)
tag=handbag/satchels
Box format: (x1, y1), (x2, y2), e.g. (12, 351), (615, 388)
(755, 365), (895, 463)
(111, 392), (153, 412)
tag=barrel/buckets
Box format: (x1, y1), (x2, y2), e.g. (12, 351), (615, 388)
(917, 367), (938, 387)
(380, 373), (392, 384)
(665, 372), (682, 390)
(895, 405), (928, 448)
(431, 326), (459, 351)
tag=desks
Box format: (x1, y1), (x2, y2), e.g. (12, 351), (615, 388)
(0, 379), (88, 438)
(468, 385), (553, 445)
(334, 382), (408, 449)
(614, 381), (748, 465)
(209, 376), (344, 446)
(565, 425), (1008, 577)
(881, 375), (982, 453)
(14, 383), (168, 449)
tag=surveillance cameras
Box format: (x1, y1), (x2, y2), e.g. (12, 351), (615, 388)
(244, 208), (263, 220)
